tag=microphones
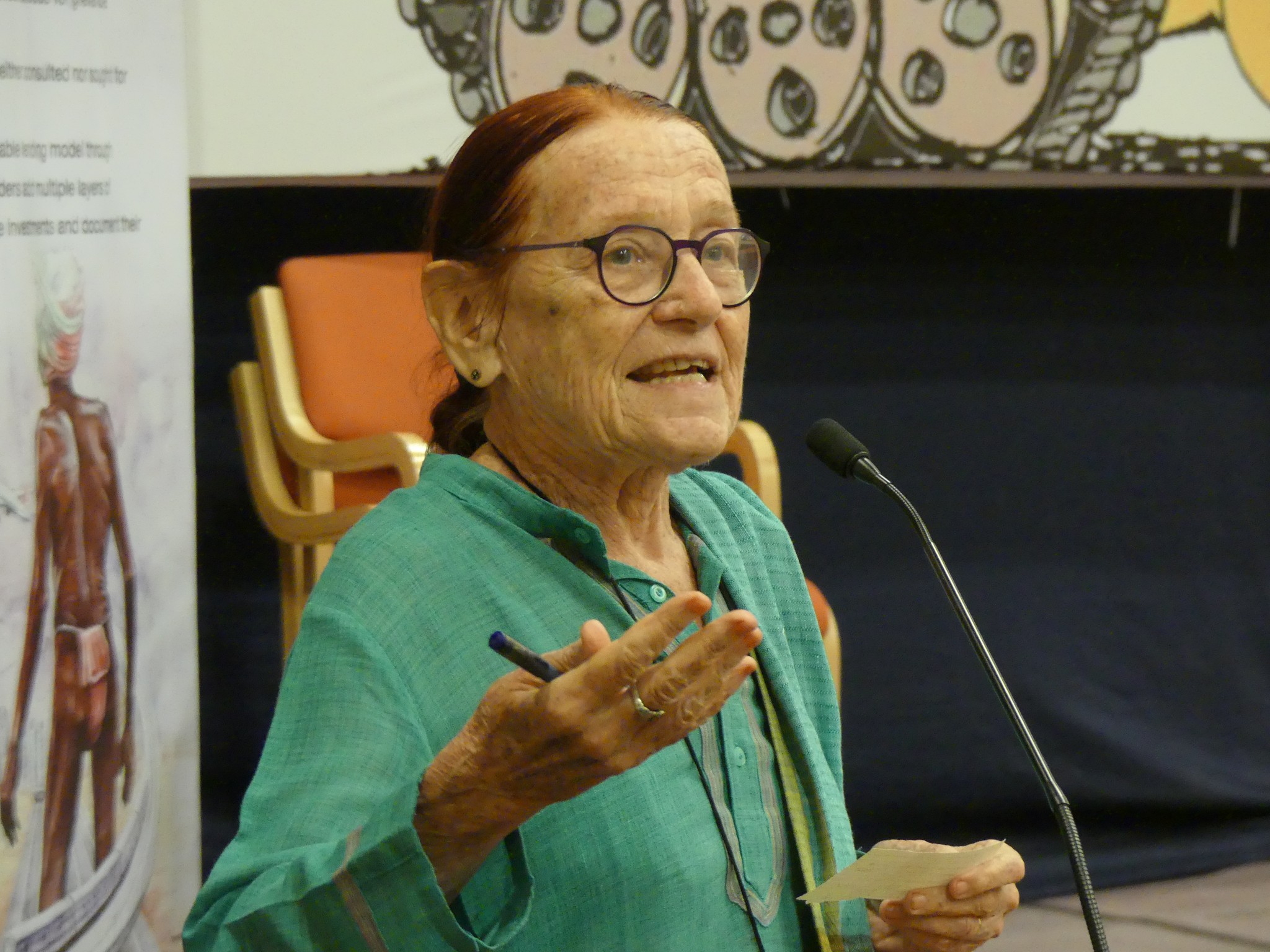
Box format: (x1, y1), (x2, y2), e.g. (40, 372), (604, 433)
(803, 418), (1110, 952)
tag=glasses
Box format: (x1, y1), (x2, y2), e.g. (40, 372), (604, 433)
(479, 222), (769, 309)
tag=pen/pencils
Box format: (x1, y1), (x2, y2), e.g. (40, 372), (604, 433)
(488, 627), (564, 685)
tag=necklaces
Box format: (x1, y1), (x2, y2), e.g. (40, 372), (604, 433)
(487, 439), (764, 952)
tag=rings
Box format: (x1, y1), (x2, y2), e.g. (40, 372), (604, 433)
(629, 685), (665, 720)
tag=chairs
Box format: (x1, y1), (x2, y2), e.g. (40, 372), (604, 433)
(231, 249), (844, 703)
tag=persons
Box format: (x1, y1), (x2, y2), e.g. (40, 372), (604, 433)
(182, 81), (1025, 952)
